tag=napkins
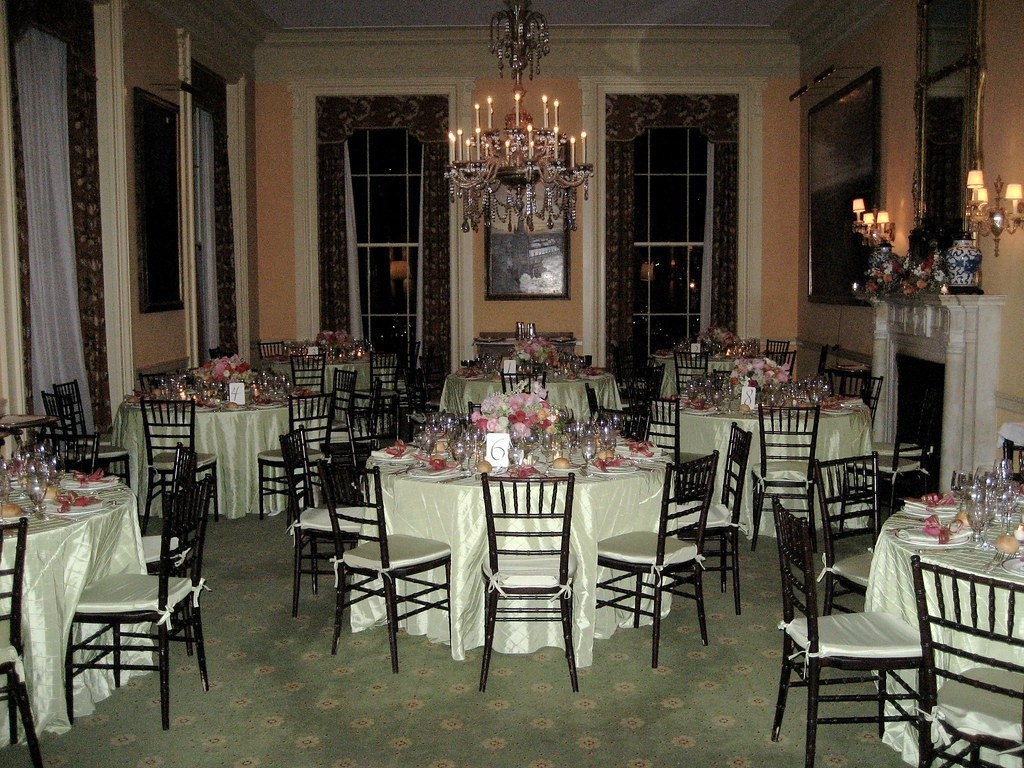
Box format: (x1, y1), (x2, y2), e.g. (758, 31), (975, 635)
(589, 457), (634, 470)
(617, 444), (668, 456)
(893, 525), (973, 543)
(409, 460), (464, 477)
(904, 498), (959, 515)
(368, 445), (420, 459)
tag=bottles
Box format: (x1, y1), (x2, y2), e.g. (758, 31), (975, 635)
(0, 439), (67, 520)
(283, 332), (372, 361)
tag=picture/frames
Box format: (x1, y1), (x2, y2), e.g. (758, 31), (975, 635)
(913, 0), (987, 296)
(134, 87), (184, 313)
(484, 140), (571, 300)
(808, 66), (882, 306)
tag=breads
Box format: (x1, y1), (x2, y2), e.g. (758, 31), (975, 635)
(44, 486), (61, 499)
(0, 502), (22, 518)
(127, 389), (238, 409)
(995, 535), (1020, 553)
(798, 399), (840, 411)
(739, 404), (750, 412)
(431, 442), (614, 473)
(955, 510), (972, 526)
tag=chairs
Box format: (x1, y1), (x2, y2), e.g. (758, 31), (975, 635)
(0, 339), (1023, 768)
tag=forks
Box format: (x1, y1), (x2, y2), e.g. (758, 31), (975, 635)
(984, 551), (1017, 573)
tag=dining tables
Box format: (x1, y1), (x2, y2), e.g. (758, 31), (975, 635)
(350, 436), (677, 669)
(260, 352), (397, 423)
(0, 472), (154, 748)
(863, 487), (1024, 768)
(646, 354), (766, 399)
(109, 391), (328, 520)
(439, 370), (624, 421)
(644, 396), (873, 537)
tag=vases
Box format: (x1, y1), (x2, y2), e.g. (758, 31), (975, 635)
(945, 240), (983, 287)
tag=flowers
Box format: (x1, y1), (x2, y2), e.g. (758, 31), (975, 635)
(317, 330), (353, 347)
(467, 393), (561, 439)
(187, 354), (258, 382)
(710, 327), (736, 349)
(732, 357), (791, 393)
(512, 335), (557, 368)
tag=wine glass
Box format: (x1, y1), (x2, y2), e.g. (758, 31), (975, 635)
(414, 401), (619, 472)
(951, 457), (1018, 546)
(124, 354), (311, 412)
(456, 343), (588, 382)
(666, 328), (839, 412)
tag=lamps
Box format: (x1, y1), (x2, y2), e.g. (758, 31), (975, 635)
(443, 0), (594, 233)
(852, 198), (895, 248)
(965, 170), (1024, 257)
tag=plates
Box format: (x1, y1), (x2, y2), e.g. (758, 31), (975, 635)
(548, 431), (665, 475)
(371, 447), (417, 462)
(896, 527), (972, 548)
(901, 500), (959, 520)
(672, 395), (861, 419)
(0, 511), (31, 523)
(40, 497), (103, 516)
(407, 468), (461, 477)
(65, 475), (119, 491)
(1001, 557), (1024, 577)
